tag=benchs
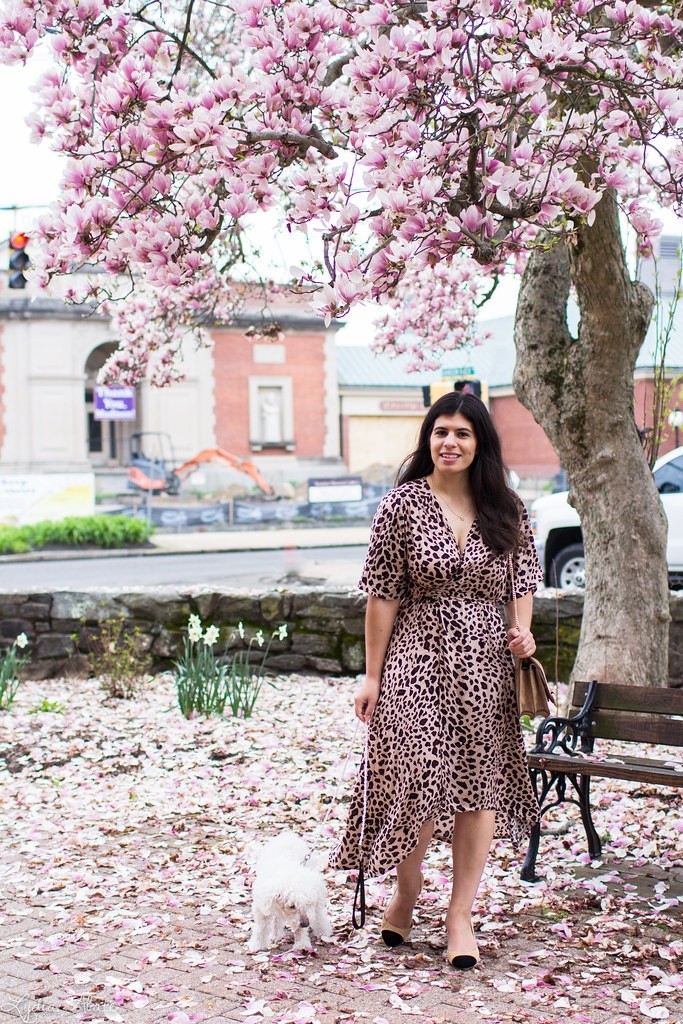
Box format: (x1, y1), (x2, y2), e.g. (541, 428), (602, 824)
(519, 681), (682, 882)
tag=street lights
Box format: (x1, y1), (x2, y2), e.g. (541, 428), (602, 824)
(668, 406), (683, 448)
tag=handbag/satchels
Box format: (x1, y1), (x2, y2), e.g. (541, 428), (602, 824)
(515, 655), (557, 720)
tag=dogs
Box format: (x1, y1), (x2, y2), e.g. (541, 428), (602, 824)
(245, 829), (332, 955)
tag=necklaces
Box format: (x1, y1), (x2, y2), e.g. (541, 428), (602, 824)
(432, 482), (472, 521)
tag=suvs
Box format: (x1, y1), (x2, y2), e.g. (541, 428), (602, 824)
(527, 445), (683, 590)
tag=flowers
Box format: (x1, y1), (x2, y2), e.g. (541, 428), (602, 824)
(170, 614), (288, 719)
(0, 632), (35, 712)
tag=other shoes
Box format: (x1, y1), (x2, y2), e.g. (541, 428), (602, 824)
(380, 872), (424, 947)
(444, 915), (480, 967)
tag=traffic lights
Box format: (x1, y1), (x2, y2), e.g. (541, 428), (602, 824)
(454, 377), (482, 399)
(9, 232), (30, 288)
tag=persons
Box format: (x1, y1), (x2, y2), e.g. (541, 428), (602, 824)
(328, 392), (544, 970)
